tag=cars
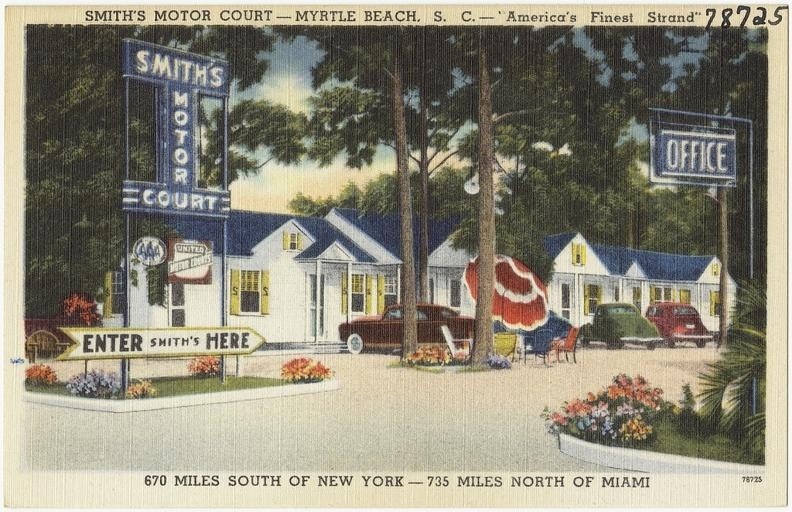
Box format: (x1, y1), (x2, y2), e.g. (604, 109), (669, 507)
(576, 303), (664, 350)
(339, 302), (477, 355)
(646, 302), (714, 349)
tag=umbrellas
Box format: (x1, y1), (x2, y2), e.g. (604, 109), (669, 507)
(462, 253), (549, 333)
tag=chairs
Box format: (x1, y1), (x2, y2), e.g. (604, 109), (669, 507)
(440, 324), (581, 368)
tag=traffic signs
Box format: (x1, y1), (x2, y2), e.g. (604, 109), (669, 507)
(56, 325), (264, 361)
